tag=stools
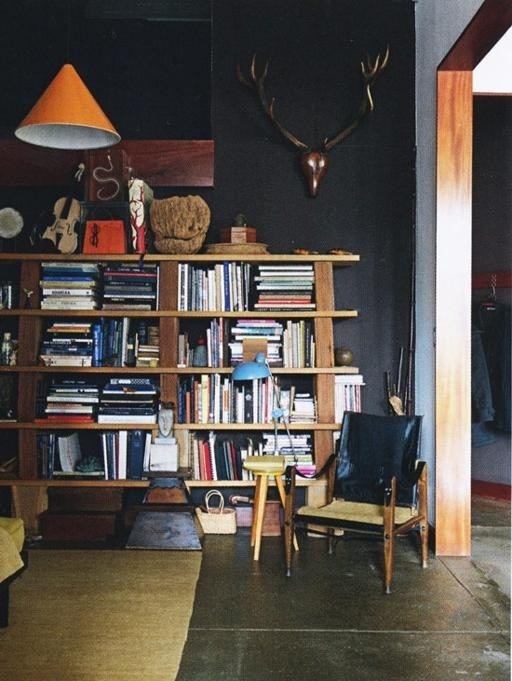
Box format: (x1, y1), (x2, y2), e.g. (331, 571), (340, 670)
(241, 452), (301, 561)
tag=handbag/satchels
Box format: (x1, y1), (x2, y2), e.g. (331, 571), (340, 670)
(194, 490), (236, 534)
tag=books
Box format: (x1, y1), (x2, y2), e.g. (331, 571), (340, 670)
(334, 374), (363, 424)
(174, 263), (318, 482)
(40, 261), (160, 480)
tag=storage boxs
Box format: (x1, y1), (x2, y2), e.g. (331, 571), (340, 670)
(36, 485), (124, 548)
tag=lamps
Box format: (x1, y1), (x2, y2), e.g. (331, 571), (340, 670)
(12, 0), (123, 151)
(230, 350), (301, 461)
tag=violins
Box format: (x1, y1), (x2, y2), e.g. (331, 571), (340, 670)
(40, 164), (85, 255)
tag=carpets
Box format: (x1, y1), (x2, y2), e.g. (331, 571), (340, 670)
(1, 545), (204, 680)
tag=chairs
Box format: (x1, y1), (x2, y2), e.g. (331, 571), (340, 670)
(282, 409), (429, 596)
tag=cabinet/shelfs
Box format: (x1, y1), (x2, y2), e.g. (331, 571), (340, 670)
(0, 253), (362, 545)
